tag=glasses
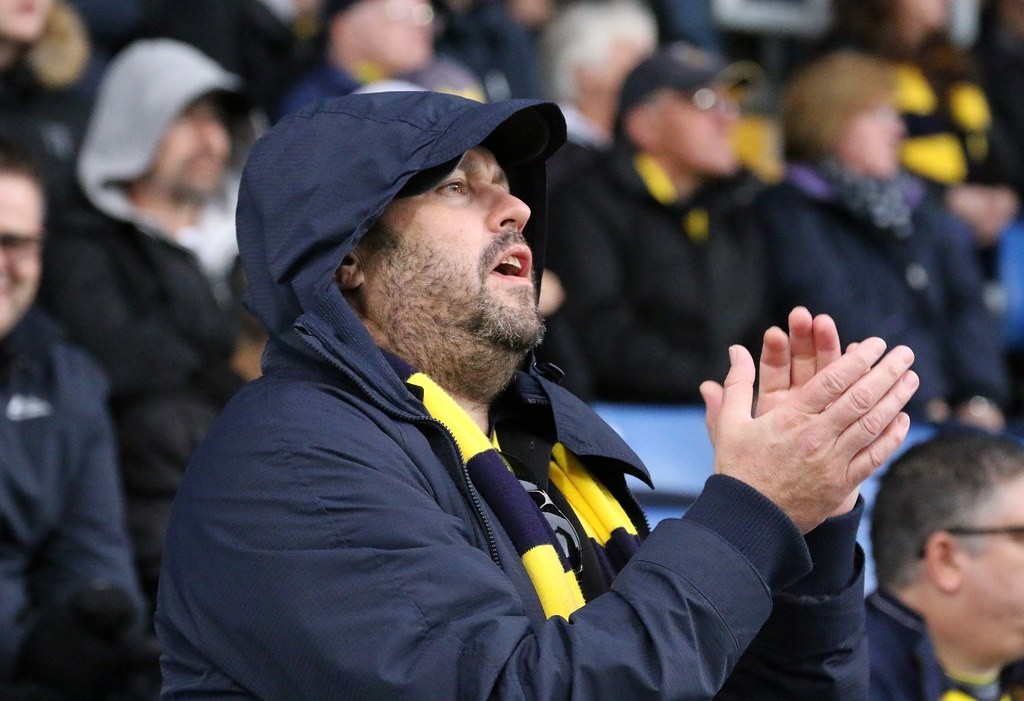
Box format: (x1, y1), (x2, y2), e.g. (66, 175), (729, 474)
(919, 522), (1024, 559)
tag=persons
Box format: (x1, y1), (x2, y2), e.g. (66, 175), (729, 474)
(0, 165), (150, 701)
(0, 0), (1024, 421)
(156, 91), (920, 701)
(864, 426), (1024, 701)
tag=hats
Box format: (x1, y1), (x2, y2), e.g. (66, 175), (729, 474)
(617, 41), (770, 105)
